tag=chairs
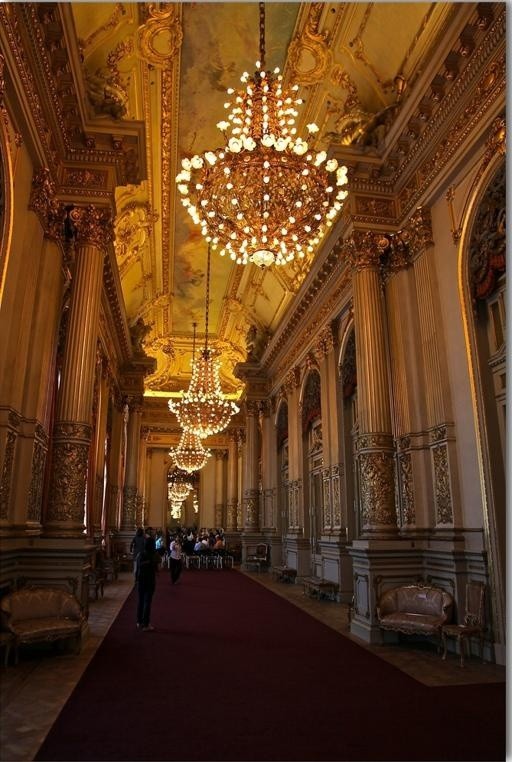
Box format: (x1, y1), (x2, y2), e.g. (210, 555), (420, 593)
(441, 583), (488, 668)
(245, 543), (268, 576)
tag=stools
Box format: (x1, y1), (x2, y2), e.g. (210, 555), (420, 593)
(183, 550), (234, 570)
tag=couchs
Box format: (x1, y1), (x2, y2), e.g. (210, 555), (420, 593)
(0, 584), (85, 667)
(377, 583), (452, 656)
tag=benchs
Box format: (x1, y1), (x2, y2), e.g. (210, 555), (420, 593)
(272, 566), (297, 586)
(303, 576), (338, 602)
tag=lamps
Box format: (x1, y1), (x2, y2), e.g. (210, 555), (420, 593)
(165, 242), (240, 501)
(172, 1), (350, 271)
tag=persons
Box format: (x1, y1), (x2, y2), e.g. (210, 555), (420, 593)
(132, 536), (163, 631)
(129, 523), (229, 585)
